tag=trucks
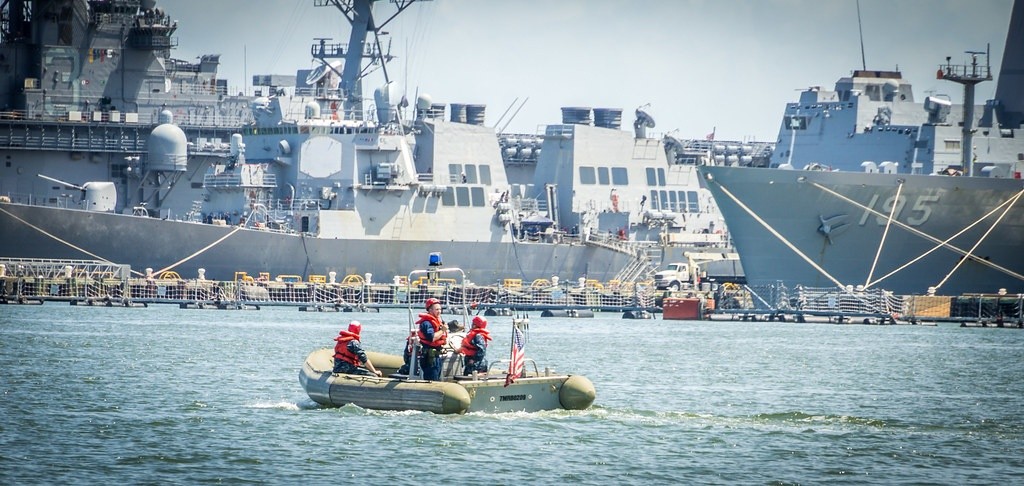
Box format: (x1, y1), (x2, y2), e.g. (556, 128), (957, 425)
(654, 262), (748, 291)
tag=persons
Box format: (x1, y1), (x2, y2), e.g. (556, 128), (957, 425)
(397, 297), (463, 381)
(333, 320), (384, 377)
(455, 316), (493, 375)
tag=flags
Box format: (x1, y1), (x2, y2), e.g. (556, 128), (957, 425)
(504, 324), (526, 386)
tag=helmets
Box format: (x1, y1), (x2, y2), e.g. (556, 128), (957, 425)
(348, 321), (361, 333)
(472, 316), (487, 328)
(425, 298), (440, 309)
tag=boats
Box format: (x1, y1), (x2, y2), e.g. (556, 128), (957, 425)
(0, 0), (770, 309)
(697, 0), (1024, 323)
(299, 268), (597, 415)
